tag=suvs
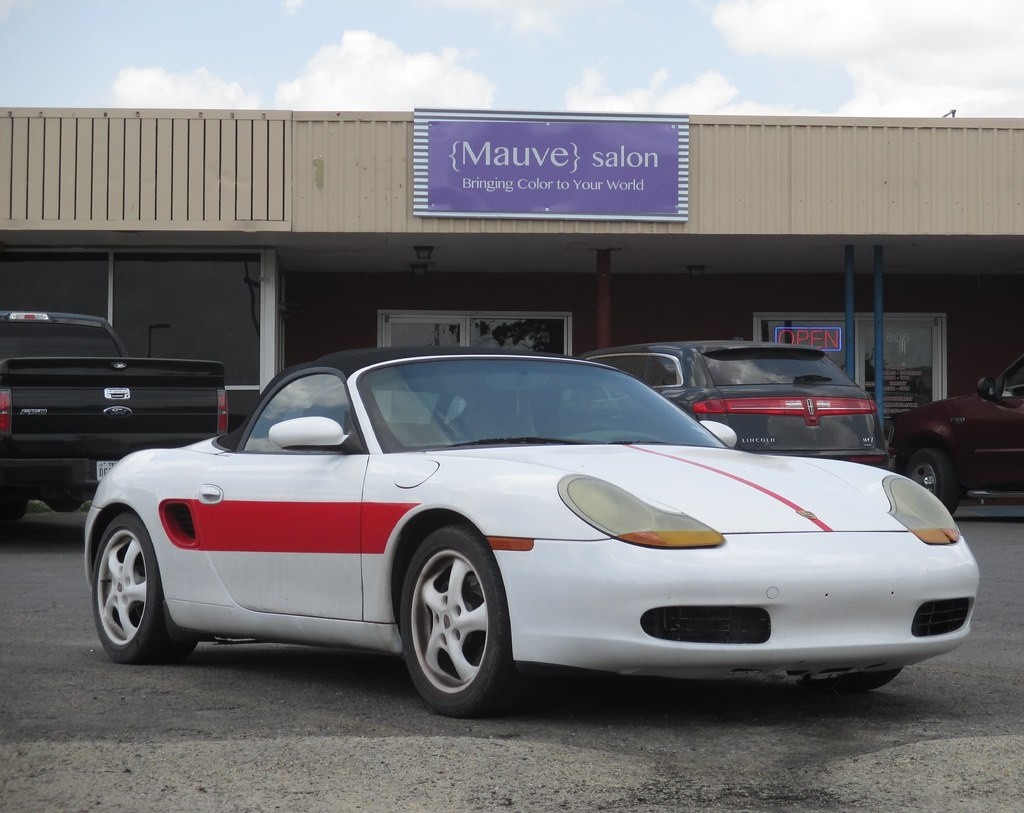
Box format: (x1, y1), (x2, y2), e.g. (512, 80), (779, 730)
(891, 350), (1024, 518)
(528, 341), (892, 470)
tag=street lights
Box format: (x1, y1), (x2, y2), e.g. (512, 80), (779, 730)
(146, 322), (170, 357)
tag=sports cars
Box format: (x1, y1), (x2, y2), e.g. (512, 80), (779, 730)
(80, 341), (982, 717)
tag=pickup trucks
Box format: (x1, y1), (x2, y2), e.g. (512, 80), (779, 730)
(0, 308), (230, 522)
